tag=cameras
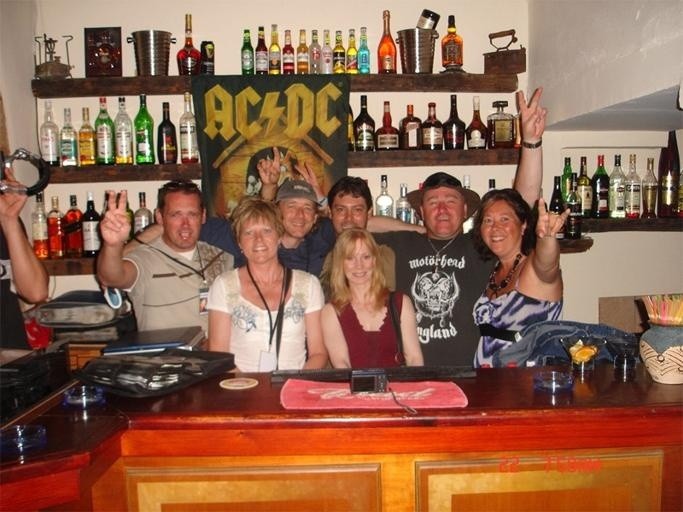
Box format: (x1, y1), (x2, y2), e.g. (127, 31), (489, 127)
(349, 366), (387, 395)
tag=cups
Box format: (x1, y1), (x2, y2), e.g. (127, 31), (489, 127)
(562, 337), (605, 373)
(63, 385), (103, 409)
(0, 424), (47, 459)
(604, 336), (639, 371)
(532, 371), (574, 393)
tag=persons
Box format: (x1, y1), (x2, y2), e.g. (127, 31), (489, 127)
(95, 177), (235, 351)
(0, 167), (49, 351)
(204, 196), (329, 373)
(293, 86), (547, 365)
(256, 146), (373, 238)
(319, 227), (424, 369)
(122, 179), (426, 280)
(471, 188), (571, 370)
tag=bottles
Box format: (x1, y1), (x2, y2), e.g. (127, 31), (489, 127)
(29, 192), (152, 260)
(547, 128), (683, 238)
(440, 15), (464, 69)
(415, 8), (440, 30)
(637, 319), (683, 387)
(347, 93), (518, 152)
(38, 92), (198, 167)
(176, 14), (201, 74)
(240, 24), (371, 74)
(462, 175), (474, 234)
(377, 9), (396, 76)
(487, 179), (495, 191)
(364, 175), (425, 228)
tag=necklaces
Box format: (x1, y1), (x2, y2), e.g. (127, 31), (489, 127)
(427, 233), (459, 273)
(488, 254), (522, 292)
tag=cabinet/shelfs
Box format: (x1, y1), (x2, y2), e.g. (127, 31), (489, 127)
(27, 74), (593, 279)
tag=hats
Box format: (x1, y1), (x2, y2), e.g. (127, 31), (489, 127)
(275, 180), (322, 206)
(404, 171), (482, 221)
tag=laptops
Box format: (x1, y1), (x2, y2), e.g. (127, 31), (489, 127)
(100, 324), (206, 357)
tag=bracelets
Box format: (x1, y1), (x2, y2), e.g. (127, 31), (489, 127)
(316, 196), (328, 209)
(521, 140), (542, 149)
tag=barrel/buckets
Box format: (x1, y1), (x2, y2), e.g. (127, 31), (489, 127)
(395, 28), (439, 74)
(126, 30), (177, 76)
